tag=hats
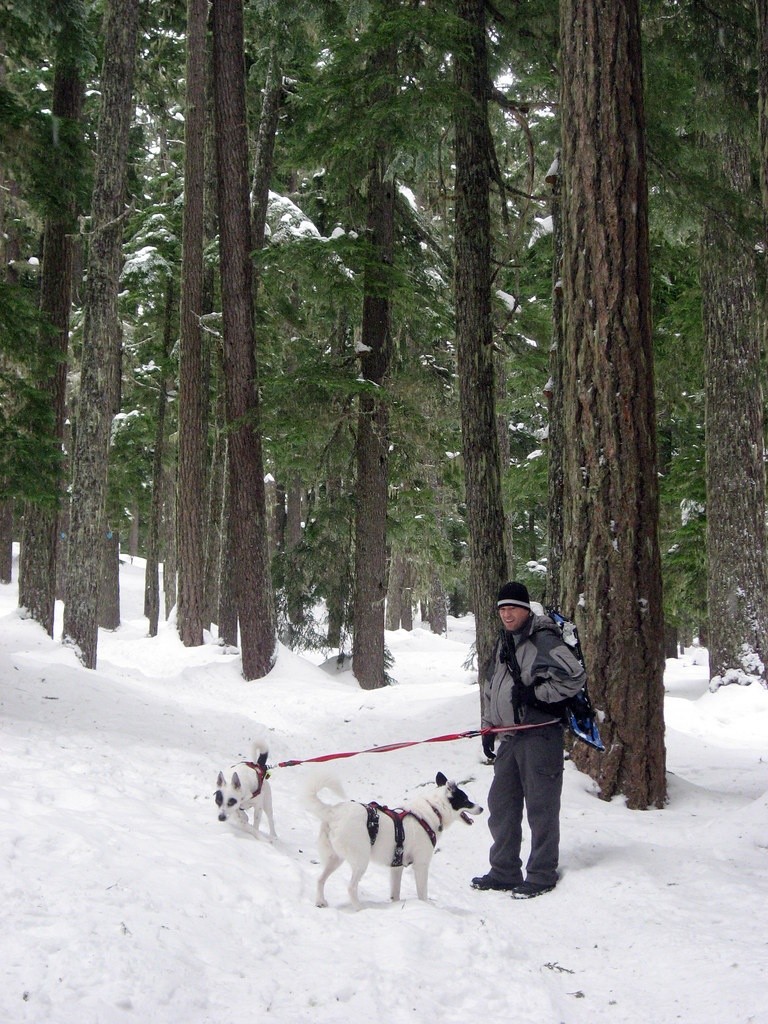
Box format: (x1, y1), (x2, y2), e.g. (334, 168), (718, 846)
(496, 582), (530, 615)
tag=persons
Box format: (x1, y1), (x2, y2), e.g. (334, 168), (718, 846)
(471, 582), (587, 899)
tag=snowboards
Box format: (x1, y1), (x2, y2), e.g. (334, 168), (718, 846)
(544, 602), (608, 753)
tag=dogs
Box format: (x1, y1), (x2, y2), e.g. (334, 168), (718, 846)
(213, 738), (278, 845)
(299, 768), (484, 910)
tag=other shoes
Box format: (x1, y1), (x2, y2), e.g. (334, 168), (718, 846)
(470, 875), (524, 890)
(512, 880), (556, 899)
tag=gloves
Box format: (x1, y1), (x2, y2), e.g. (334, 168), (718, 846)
(482, 732), (497, 759)
(511, 684), (537, 706)
